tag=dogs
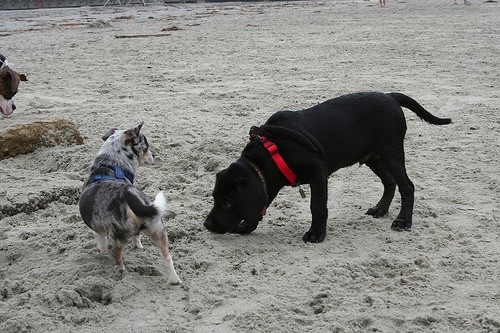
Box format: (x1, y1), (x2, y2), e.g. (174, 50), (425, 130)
(0, 52), (29, 116)
(203, 90), (452, 243)
(78, 121), (183, 285)
